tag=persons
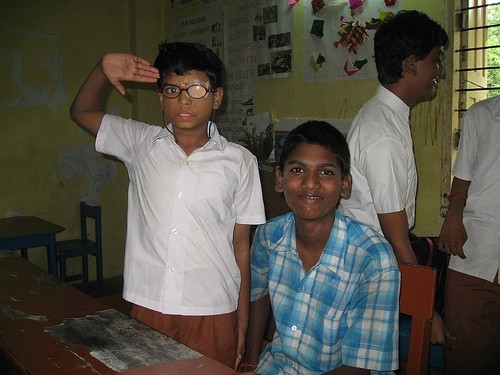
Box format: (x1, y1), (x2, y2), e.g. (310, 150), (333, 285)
(343, 11), (500, 375)
(246, 119), (403, 375)
(69, 42), (268, 372)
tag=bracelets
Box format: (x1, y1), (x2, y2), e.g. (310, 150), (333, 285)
(447, 192), (467, 201)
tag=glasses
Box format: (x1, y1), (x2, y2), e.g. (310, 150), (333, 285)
(156, 82), (217, 100)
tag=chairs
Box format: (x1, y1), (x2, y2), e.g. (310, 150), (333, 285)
(398, 261), (438, 375)
(55, 202), (103, 291)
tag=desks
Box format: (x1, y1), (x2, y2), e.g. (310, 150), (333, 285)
(0, 216), (65, 278)
